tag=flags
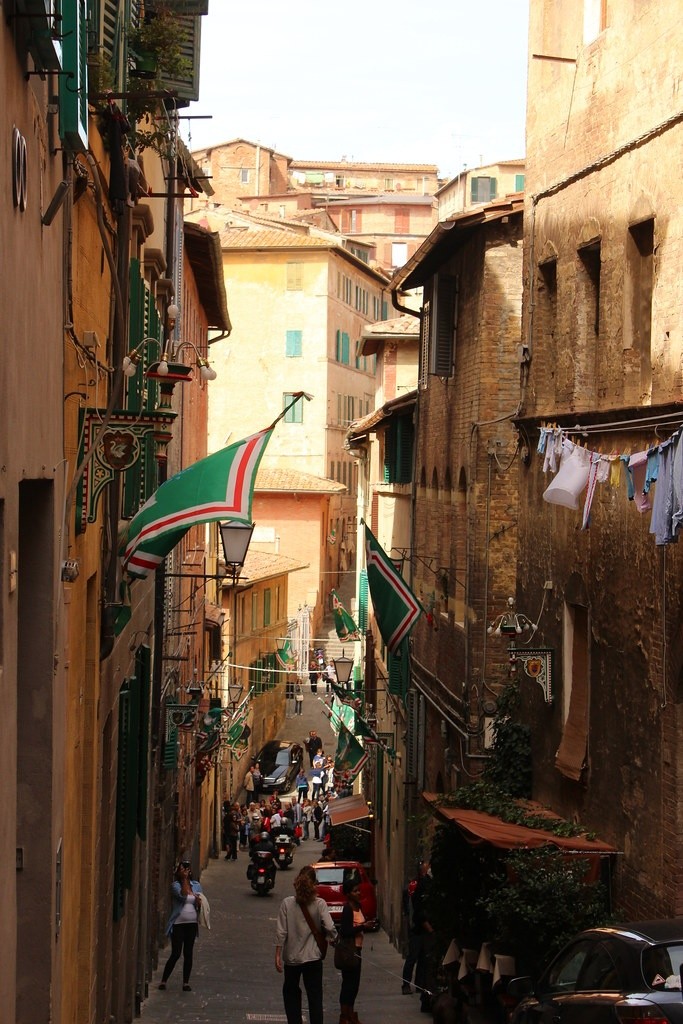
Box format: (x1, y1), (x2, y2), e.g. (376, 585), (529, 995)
(332, 697), (358, 733)
(333, 721), (369, 793)
(332, 589), (361, 642)
(365, 523), (421, 650)
(328, 712), (363, 746)
(228, 697), (250, 730)
(123, 427), (273, 580)
(196, 708), (223, 774)
(227, 707), (252, 749)
(275, 635), (298, 673)
(333, 685), (365, 719)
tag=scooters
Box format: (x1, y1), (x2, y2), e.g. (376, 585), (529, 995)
(275, 834), (294, 868)
(248, 851), (274, 895)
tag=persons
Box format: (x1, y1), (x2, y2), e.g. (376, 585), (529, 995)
(333, 880), (380, 1024)
(158, 860), (202, 989)
(223, 731), (354, 860)
(400, 869), (437, 995)
(295, 679), (303, 716)
(309, 659), (337, 694)
(274, 866), (337, 1024)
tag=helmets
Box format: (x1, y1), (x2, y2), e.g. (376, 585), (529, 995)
(281, 817), (288, 824)
(262, 832), (269, 839)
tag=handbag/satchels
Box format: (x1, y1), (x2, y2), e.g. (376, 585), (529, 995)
(334, 943), (359, 969)
(299, 903), (327, 958)
(293, 825), (302, 837)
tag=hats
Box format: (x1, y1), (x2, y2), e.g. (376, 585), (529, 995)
(253, 813), (259, 819)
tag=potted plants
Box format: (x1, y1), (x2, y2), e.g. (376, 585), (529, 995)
(120, 7), (195, 80)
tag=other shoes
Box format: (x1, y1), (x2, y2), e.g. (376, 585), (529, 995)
(403, 986), (412, 994)
(159, 985), (165, 989)
(313, 838), (324, 841)
(339, 1007), (360, 1024)
(302, 838), (308, 840)
(183, 985), (191, 990)
(224, 858), (237, 861)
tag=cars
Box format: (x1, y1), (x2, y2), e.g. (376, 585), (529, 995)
(507, 921), (683, 1024)
(251, 740), (304, 793)
(308, 860), (381, 930)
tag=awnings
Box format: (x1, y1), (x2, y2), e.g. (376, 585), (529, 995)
(329, 794), (370, 833)
(424, 791), (625, 858)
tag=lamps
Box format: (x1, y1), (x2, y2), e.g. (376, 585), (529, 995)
(123, 307), (218, 381)
(487, 597), (538, 638)
(218, 520), (256, 588)
(226, 677), (243, 712)
(330, 647), (354, 689)
